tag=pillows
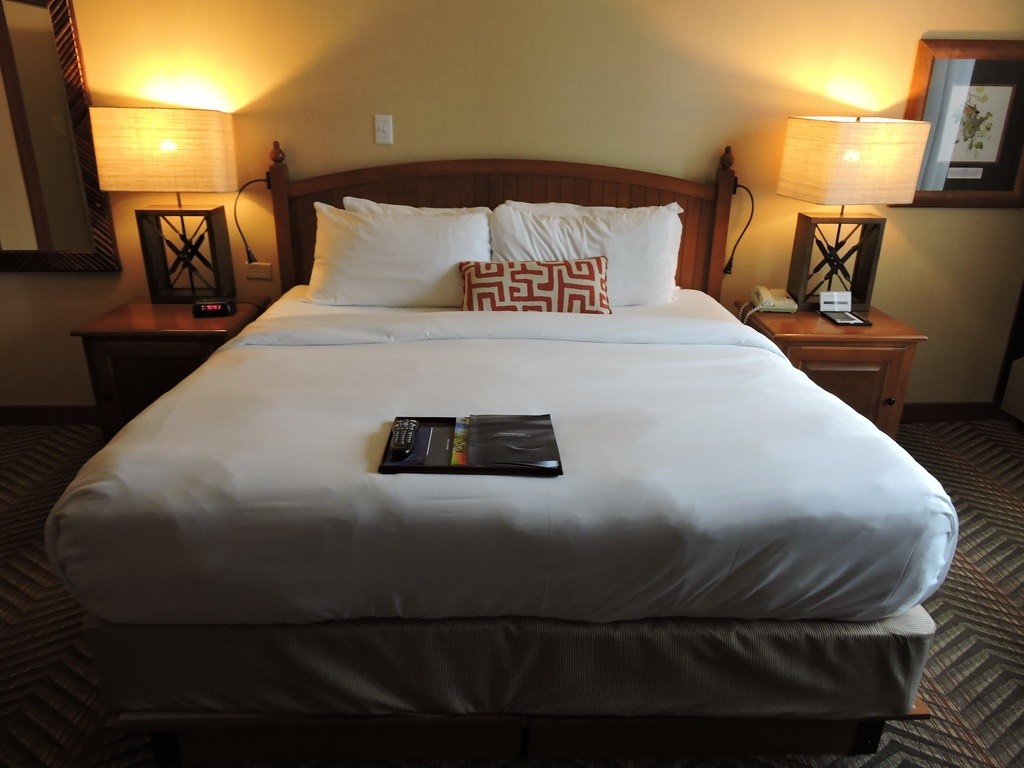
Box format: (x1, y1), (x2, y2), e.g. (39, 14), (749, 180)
(342, 197), (494, 219)
(457, 255), (613, 315)
(490, 200), (684, 309)
(303, 201), (492, 308)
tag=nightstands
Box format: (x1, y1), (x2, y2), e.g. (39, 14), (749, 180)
(735, 300), (928, 442)
(70, 295), (272, 448)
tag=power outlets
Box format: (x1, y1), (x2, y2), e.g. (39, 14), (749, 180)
(245, 262), (272, 279)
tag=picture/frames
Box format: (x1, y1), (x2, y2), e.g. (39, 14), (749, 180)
(887, 40), (1024, 209)
(0, 0), (122, 271)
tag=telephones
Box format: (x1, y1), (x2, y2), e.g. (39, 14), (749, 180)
(747, 285), (798, 314)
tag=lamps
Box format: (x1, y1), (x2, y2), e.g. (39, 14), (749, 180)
(724, 176), (754, 274)
(775, 115), (930, 311)
(235, 171), (272, 263)
(89, 107), (239, 305)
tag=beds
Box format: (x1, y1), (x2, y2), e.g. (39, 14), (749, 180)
(45, 140), (959, 757)
